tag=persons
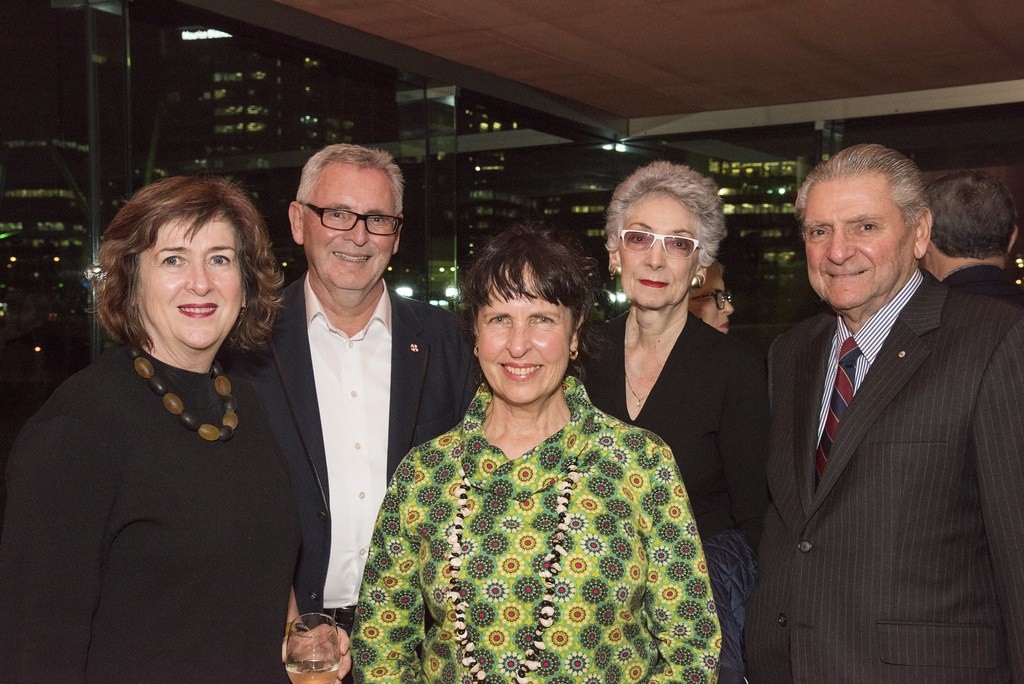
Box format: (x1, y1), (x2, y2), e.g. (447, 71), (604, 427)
(687, 260), (734, 332)
(744, 144), (1024, 684)
(916, 169), (1024, 312)
(0, 175), (353, 684)
(227, 143), (486, 684)
(349, 222), (721, 684)
(578, 159), (770, 579)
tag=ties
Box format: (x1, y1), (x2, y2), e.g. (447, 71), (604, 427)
(814, 336), (862, 494)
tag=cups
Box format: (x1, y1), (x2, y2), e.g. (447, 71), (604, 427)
(284, 612), (343, 684)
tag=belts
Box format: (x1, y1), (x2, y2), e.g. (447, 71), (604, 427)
(321, 606), (355, 627)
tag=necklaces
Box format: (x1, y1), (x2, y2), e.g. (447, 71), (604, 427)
(449, 461), (579, 684)
(625, 374), (649, 407)
(123, 339), (239, 442)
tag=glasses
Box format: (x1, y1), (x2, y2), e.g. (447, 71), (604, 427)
(620, 229), (701, 259)
(297, 202), (404, 236)
(688, 289), (732, 310)
